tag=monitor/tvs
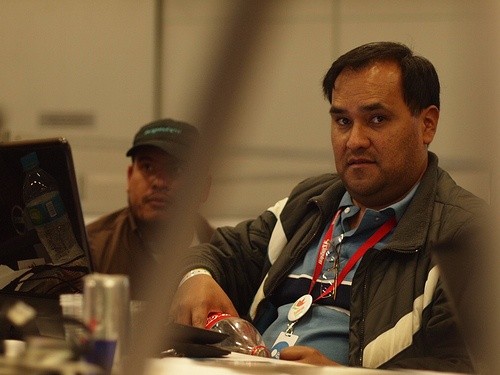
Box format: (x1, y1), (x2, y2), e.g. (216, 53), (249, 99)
(0, 138), (95, 273)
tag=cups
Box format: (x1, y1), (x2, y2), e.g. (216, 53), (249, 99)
(61, 293), (82, 339)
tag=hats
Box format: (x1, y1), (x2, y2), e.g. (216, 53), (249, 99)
(125, 119), (212, 167)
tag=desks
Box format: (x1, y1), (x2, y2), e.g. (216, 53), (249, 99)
(145, 352), (465, 375)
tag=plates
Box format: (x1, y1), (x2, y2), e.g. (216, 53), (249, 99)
(171, 325), (231, 358)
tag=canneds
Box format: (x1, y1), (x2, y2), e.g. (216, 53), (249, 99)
(81, 273), (131, 370)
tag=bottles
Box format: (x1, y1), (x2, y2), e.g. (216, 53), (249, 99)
(21, 152), (84, 264)
(205, 312), (271, 358)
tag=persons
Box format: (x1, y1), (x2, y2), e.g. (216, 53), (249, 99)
(16, 118), (222, 320)
(165, 37), (488, 373)
